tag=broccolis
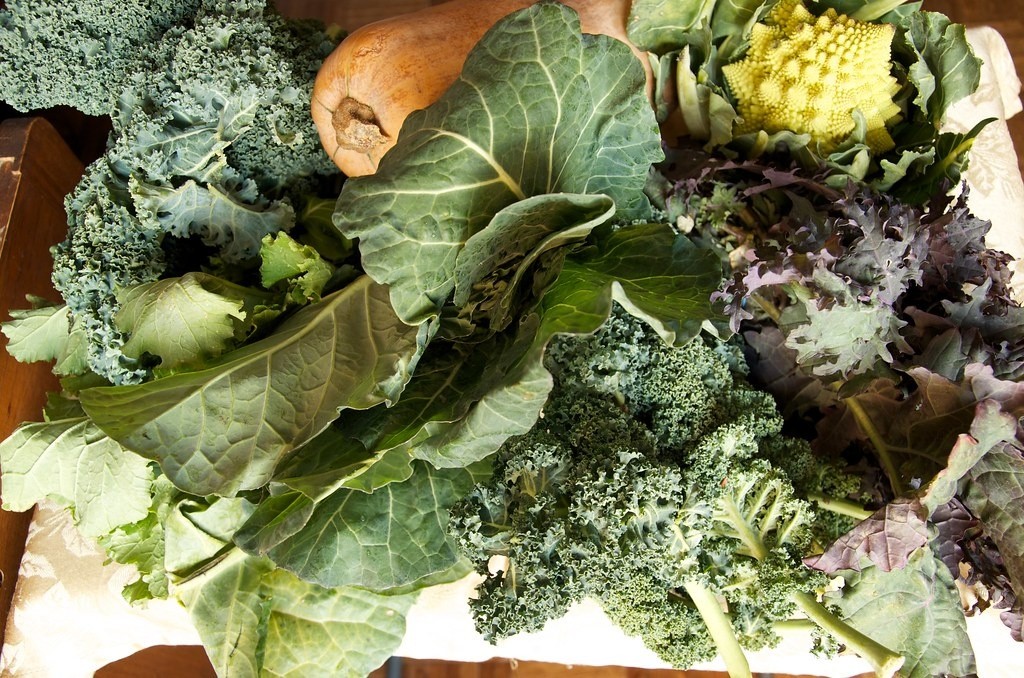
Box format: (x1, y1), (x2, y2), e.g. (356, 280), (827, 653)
(0, 0), (1024, 678)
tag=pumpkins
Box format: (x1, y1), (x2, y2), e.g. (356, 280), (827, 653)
(312, 0), (651, 178)
